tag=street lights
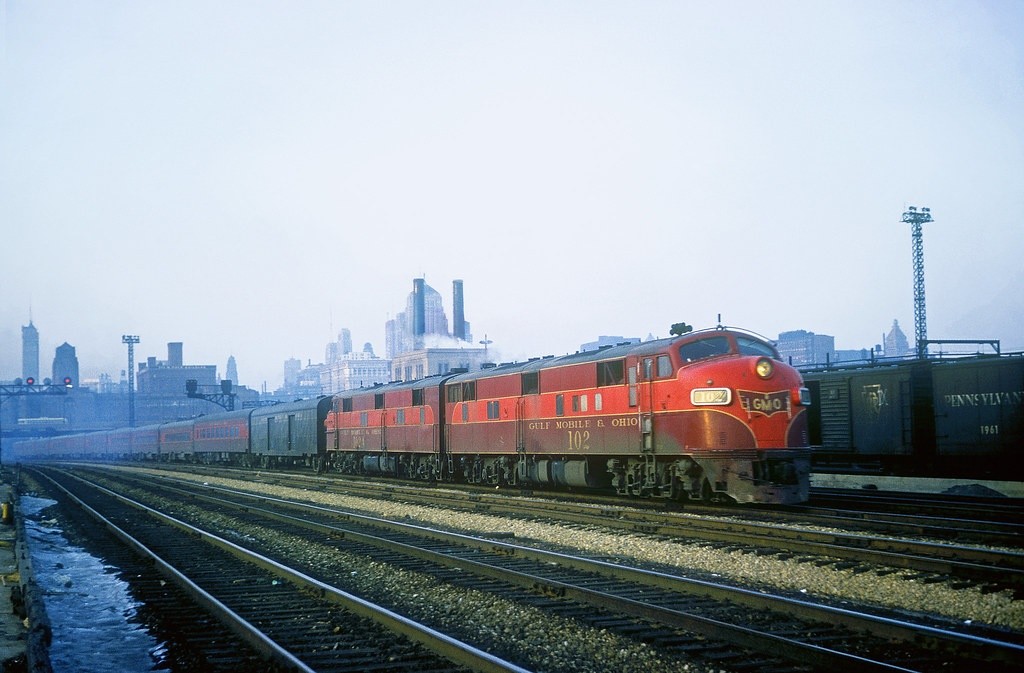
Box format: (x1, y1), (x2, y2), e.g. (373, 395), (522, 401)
(122, 335), (140, 426)
(900, 206), (934, 360)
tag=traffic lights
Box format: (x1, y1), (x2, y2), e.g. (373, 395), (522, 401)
(64, 377), (71, 385)
(27, 377), (34, 385)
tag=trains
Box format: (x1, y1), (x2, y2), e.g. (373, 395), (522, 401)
(14, 313), (824, 506)
(797, 351), (1023, 474)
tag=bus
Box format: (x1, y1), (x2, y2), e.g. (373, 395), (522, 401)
(17, 418), (68, 428)
